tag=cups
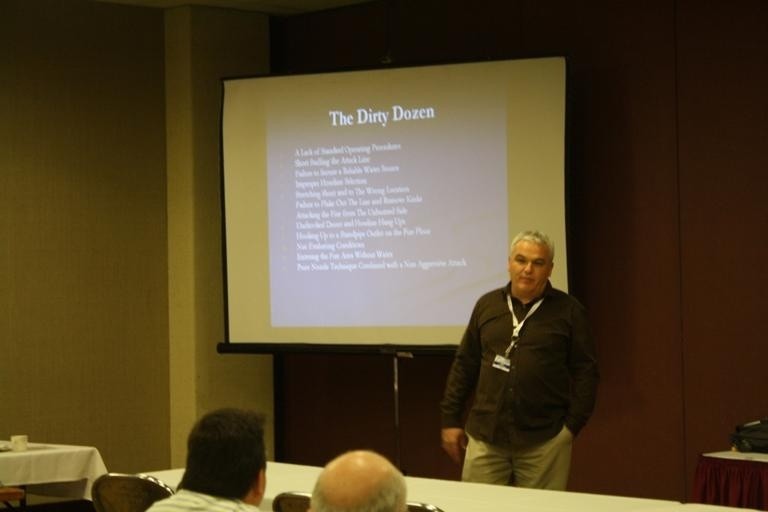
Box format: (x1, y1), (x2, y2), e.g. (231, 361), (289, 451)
(10, 435), (28, 453)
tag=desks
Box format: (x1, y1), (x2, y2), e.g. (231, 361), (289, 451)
(682, 504), (768, 512)
(0, 440), (108, 508)
(144, 462), (682, 512)
(690, 451), (768, 510)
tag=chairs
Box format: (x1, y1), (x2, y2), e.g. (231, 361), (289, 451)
(272, 493), (312, 512)
(91, 473), (175, 512)
(408, 501), (444, 512)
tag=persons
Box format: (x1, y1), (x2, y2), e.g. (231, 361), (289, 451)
(143, 407), (266, 512)
(440, 231), (598, 490)
(305, 450), (407, 512)
